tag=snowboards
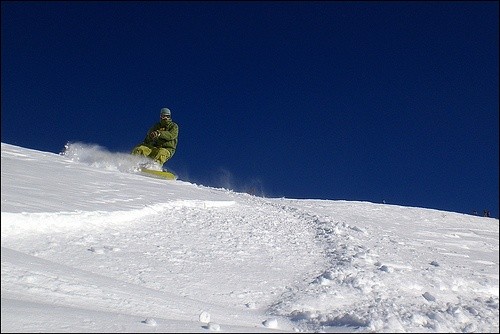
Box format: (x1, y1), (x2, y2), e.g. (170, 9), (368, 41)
(140, 166), (178, 180)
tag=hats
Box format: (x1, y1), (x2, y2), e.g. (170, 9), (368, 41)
(160, 108), (171, 115)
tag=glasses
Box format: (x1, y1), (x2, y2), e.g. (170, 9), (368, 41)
(162, 115), (170, 118)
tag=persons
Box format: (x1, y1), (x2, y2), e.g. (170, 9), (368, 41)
(130, 108), (179, 166)
